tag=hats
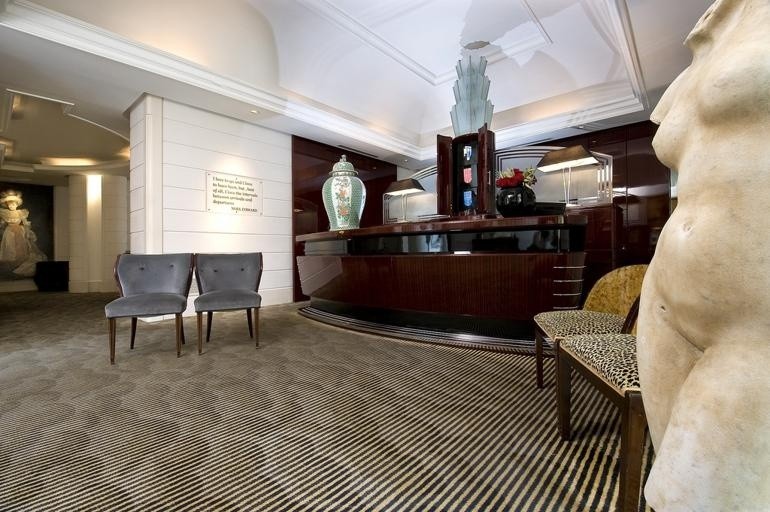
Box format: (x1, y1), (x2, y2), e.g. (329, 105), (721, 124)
(0, 189), (24, 207)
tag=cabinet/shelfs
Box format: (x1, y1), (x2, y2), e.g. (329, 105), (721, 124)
(583, 135), (670, 251)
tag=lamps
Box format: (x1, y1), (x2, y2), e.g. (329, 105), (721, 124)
(385, 178), (425, 224)
(535, 144), (598, 211)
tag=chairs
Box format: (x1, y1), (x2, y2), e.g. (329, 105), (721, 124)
(101, 249), (265, 366)
(531, 261), (657, 512)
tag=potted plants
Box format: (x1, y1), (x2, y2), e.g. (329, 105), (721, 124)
(492, 168), (538, 188)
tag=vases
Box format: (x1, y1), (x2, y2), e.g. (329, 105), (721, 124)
(496, 184), (536, 217)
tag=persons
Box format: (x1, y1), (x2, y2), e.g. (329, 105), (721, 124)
(634, 0), (770, 512)
(0, 196), (29, 262)
